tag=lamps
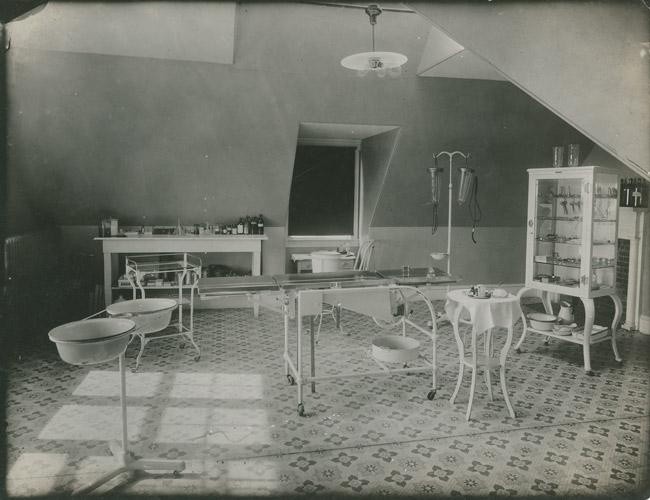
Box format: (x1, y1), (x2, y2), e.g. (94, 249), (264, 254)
(341, 5), (408, 70)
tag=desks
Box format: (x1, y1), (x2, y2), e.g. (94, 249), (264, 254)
(292, 253), (355, 274)
(443, 289), (522, 422)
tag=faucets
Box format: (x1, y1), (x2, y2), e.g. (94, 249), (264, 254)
(335, 248), (347, 254)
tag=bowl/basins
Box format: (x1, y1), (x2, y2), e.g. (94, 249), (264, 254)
(526, 313), (562, 330)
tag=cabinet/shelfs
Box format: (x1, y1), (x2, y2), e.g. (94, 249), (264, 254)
(527, 166), (620, 298)
(94, 232), (268, 318)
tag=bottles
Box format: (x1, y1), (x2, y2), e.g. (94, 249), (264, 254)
(619, 177), (649, 207)
(213, 214), (264, 235)
(568, 142), (580, 167)
(553, 143), (565, 167)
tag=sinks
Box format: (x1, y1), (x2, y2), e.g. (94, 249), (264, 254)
(308, 251), (356, 273)
